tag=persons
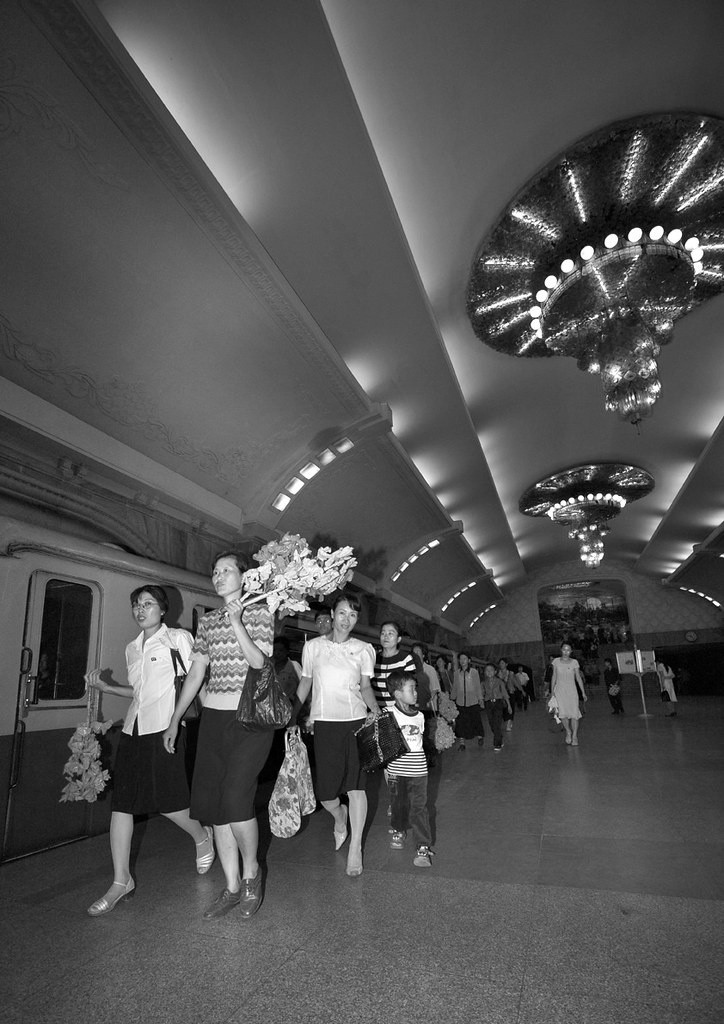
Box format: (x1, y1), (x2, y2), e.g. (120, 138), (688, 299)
(654, 655), (678, 718)
(84, 549), (534, 921)
(602, 657), (625, 716)
(543, 641), (589, 746)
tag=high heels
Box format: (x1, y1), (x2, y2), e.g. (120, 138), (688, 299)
(195, 826), (215, 874)
(87, 875), (136, 917)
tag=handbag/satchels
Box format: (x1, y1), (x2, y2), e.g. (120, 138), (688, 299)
(355, 711), (411, 774)
(427, 701), (455, 751)
(268, 725), (317, 838)
(170, 649), (199, 720)
(609, 684), (623, 696)
(236, 655), (291, 733)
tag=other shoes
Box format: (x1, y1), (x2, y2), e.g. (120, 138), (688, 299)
(566, 731), (571, 744)
(334, 804), (347, 850)
(621, 708), (624, 713)
(572, 736), (579, 745)
(346, 847), (363, 876)
(390, 831), (407, 849)
(414, 846), (432, 867)
(458, 724), (512, 751)
(611, 710), (620, 715)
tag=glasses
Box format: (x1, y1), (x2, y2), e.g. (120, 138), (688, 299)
(130, 602), (159, 609)
(315, 619), (331, 624)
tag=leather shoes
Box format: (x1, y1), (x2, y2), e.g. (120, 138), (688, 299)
(240, 866), (262, 918)
(205, 889), (240, 917)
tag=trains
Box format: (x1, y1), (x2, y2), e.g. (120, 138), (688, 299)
(1, 512), (504, 867)
